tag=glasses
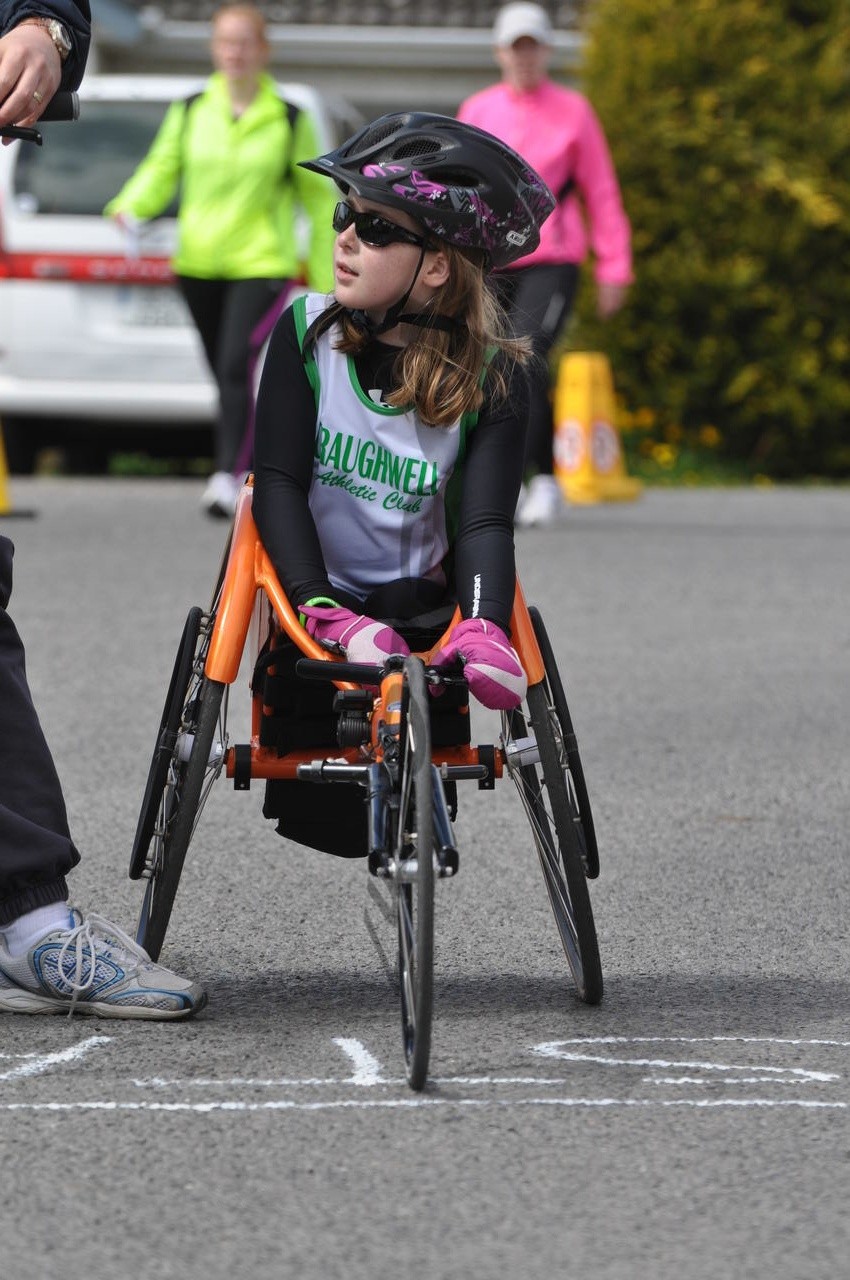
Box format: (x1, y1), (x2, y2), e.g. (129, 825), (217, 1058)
(332, 200), (441, 252)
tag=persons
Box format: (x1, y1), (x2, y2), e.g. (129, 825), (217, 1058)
(453, 1), (635, 527)
(252, 111), (529, 757)
(102, 5), (341, 521)
(0, 0), (207, 1019)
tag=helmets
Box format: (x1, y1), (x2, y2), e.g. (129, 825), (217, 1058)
(295, 111), (557, 267)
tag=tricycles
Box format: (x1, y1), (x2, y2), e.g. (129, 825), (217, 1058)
(130, 471), (603, 1091)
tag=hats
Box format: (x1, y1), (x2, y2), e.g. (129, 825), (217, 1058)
(494, 1), (551, 48)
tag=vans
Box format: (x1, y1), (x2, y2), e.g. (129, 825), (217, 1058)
(0, 74), (366, 481)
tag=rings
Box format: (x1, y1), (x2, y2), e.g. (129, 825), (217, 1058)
(33, 91), (44, 105)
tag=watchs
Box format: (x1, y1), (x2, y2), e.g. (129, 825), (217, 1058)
(18, 17), (72, 60)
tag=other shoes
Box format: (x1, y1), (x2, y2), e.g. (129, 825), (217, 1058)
(204, 470), (236, 518)
(521, 475), (558, 525)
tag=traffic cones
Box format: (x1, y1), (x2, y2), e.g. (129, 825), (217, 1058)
(548, 351), (640, 511)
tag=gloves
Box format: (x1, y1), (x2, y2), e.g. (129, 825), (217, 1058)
(298, 606), (410, 695)
(430, 618), (527, 711)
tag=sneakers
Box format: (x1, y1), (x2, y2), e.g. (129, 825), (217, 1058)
(0, 902), (208, 1019)
